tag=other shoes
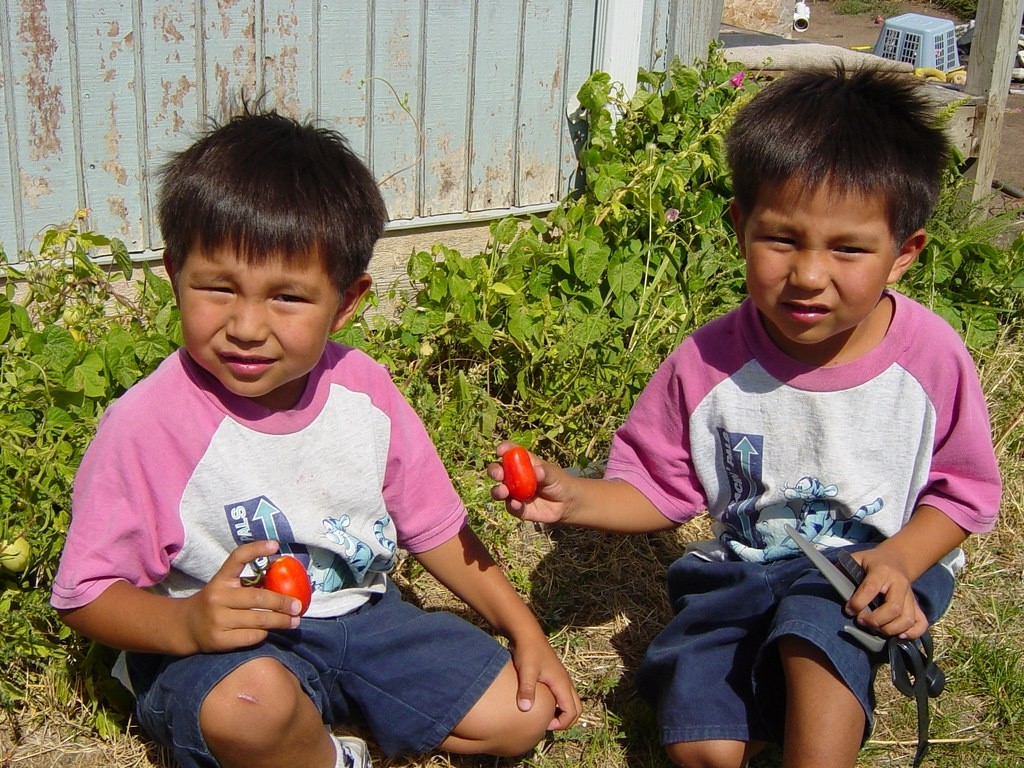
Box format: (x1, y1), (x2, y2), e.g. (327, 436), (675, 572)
(337, 735), (372, 768)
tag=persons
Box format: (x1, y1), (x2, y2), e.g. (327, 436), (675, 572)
(488, 65), (1002, 768)
(48, 113), (584, 768)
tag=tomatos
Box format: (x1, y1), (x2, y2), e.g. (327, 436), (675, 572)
(501, 447), (537, 502)
(263, 555), (312, 616)
